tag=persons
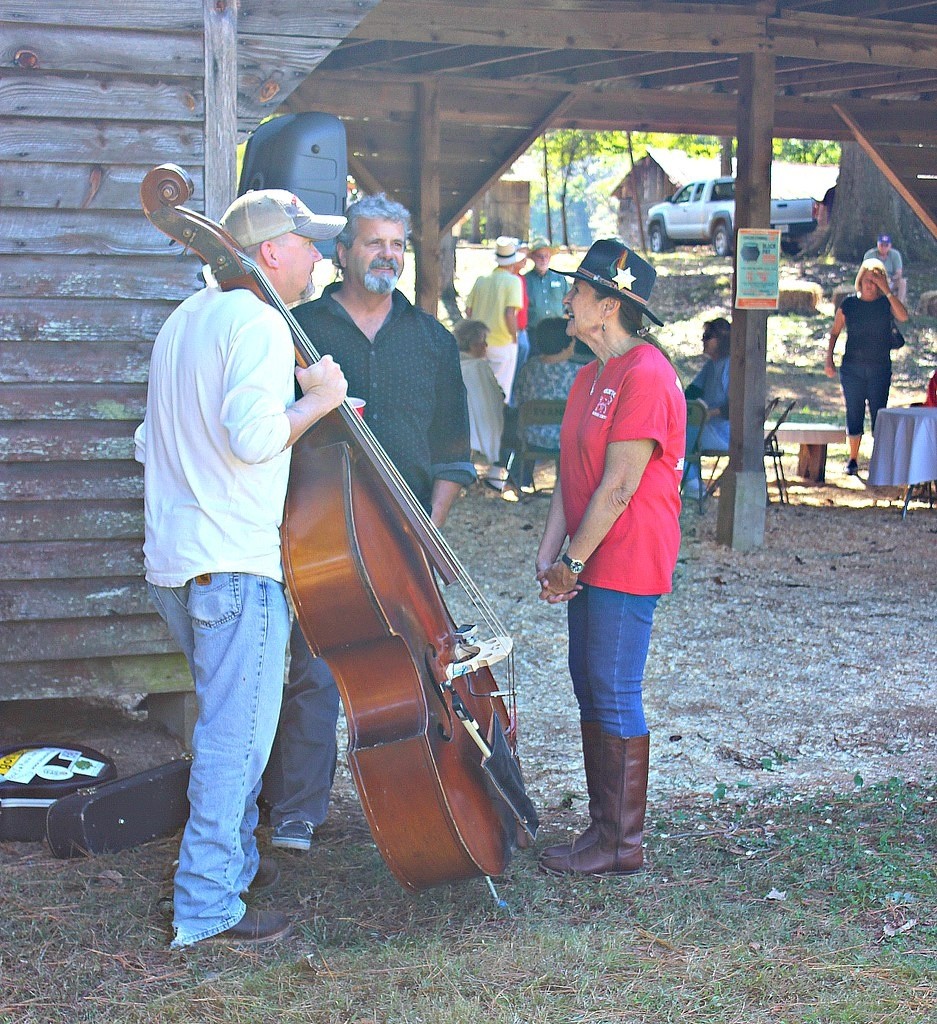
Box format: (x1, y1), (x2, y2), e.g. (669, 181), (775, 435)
(450, 235), (911, 502)
(262, 197), (477, 851)
(536, 241), (688, 876)
(134, 188), (350, 949)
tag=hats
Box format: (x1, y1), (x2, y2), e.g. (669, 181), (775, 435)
(525, 237), (560, 258)
(549, 239), (665, 327)
(511, 237), (530, 250)
(494, 235), (527, 266)
(219, 188), (347, 249)
(877, 234), (891, 245)
(703, 318), (732, 339)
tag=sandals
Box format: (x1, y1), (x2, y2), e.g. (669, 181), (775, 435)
(483, 460), (510, 492)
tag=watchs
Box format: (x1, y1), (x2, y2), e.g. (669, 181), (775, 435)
(561, 553), (585, 574)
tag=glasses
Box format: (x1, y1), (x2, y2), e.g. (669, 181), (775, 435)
(703, 333), (713, 340)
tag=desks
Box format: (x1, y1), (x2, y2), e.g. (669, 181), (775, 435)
(867, 407), (937, 517)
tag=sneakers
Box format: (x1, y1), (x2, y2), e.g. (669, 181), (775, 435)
(172, 900), (294, 947)
(271, 819), (315, 850)
(241, 855), (282, 888)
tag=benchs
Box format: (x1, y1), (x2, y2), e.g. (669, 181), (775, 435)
(763, 422), (845, 482)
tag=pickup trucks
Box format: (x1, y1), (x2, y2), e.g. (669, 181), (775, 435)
(646, 177), (818, 257)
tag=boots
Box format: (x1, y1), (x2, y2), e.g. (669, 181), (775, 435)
(539, 721), (650, 876)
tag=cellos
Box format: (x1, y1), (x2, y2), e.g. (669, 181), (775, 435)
(135, 154), (542, 912)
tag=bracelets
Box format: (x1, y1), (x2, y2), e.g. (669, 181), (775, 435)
(885, 292), (894, 297)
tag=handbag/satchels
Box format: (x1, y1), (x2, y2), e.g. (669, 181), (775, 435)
(884, 295), (905, 350)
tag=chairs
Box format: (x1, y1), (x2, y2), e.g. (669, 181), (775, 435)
(509, 399), (569, 502)
(671, 396), (798, 513)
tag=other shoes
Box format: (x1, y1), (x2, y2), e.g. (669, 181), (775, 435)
(681, 481), (706, 499)
(519, 477), (536, 494)
(845, 458), (859, 474)
(499, 480), (520, 502)
